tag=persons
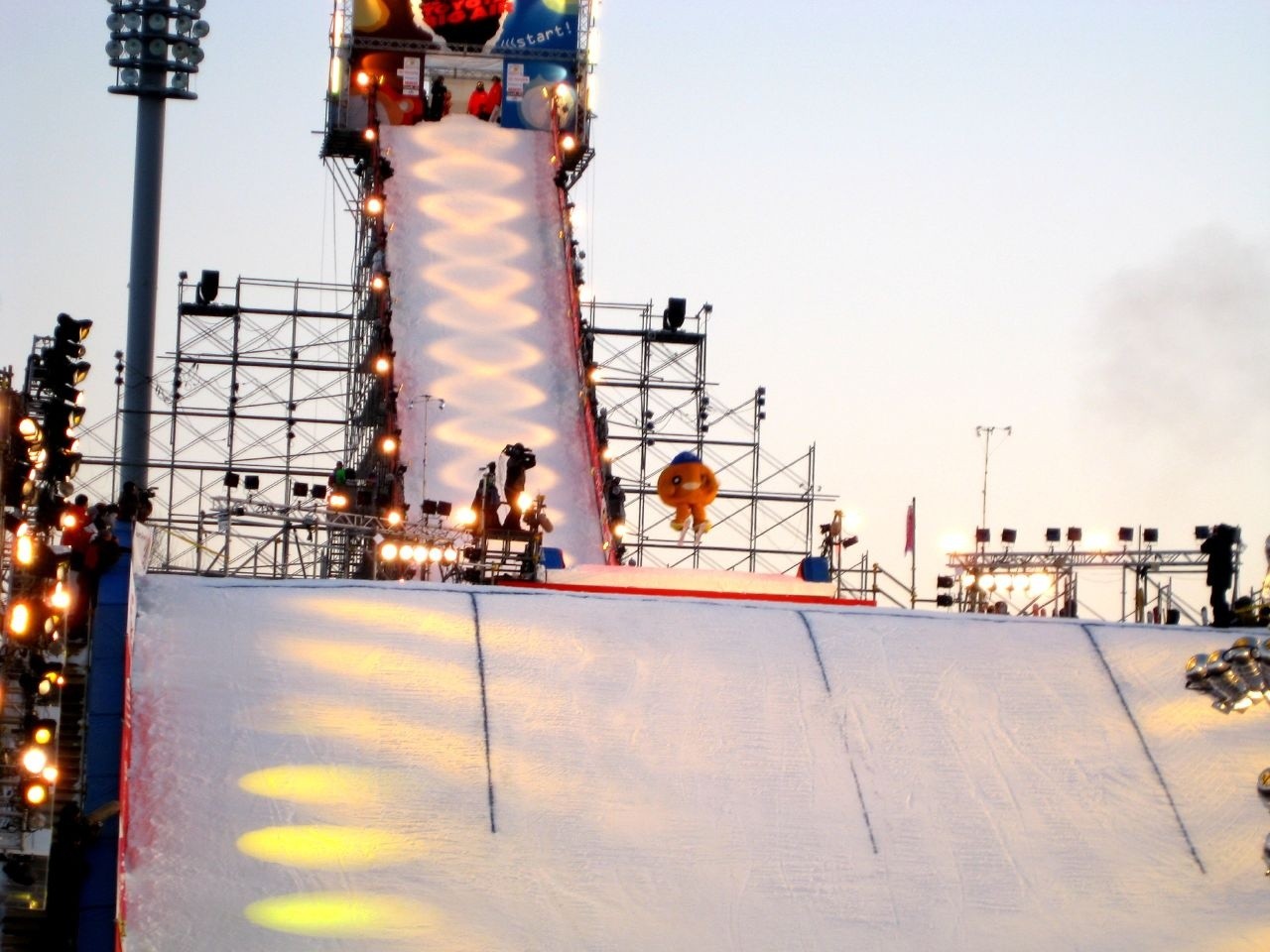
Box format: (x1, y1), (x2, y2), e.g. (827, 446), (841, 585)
(60, 493), (119, 647)
(1198, 523), (1238, 626)
(1228, 596), (1270, 628)
(470, 472), (502, 528)
(328, 460), (395, 516)
(501, 443), (536, 529)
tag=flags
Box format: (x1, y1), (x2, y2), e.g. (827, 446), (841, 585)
(905, 505), (915, 557)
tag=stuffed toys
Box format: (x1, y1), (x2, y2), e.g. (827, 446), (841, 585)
(655, 451), (720, 535)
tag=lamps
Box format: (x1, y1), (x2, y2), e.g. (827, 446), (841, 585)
(1068, 529), (1081, 540)
(936, 576), (953, 606)
(820, 524), (831, 533)
(195, 269), (219, 306)
(292, 125), (480, 563)
(4, 312), (158, 809)
(1144, 529), (1157, 541)
(1195, 526), (1209, 539)
(844, 537), (858, 548)
(1119, 528), (1133, 540)
(236, 508), (245, 516)
(1002, 530), (1016, 542)
(977, 529), (990, 542)
(663, 297), (687, 333)
(1047, 529), (1060, 540)
(538, 514), (553, 533)
(224, 472), (259, 489)
